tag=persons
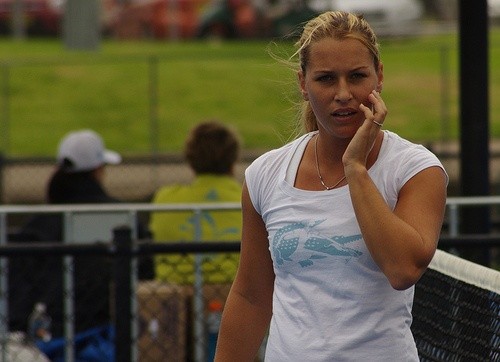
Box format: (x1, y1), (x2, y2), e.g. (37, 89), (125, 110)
(152, 121), (242, 282)
(214, 10), (449, 362)
(13, 130), (151, 326)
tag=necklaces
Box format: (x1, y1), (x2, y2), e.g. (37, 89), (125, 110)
(315, 131), (378, 190)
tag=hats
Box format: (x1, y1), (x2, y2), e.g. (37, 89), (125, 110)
(59, 130), (121, 171)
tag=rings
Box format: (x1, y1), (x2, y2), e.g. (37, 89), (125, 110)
(373, 119), (383, 127)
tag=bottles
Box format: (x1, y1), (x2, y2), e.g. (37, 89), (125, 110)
(205, 301), (223, 362)
(28, 304), (48, 338)
